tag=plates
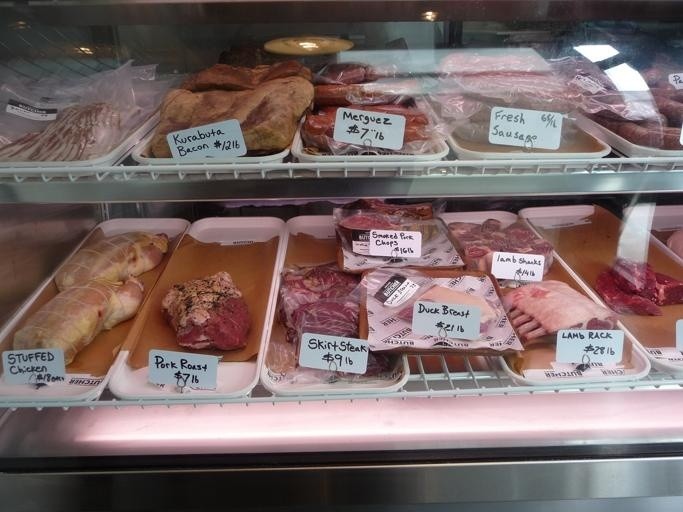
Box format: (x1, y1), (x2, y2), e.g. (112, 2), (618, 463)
(422, 86), (612, 168)
(572, 110), (683, 167)
(109, 216), (284, 401)
(358, 264), (520, 356)
(622, 204), (683, 258)
(334, 217), (470, 275)
(441, 210), (651, 385)
(130, 121), (290, 170)
(519, 204), (683, 374)
(291, 65), (450, 169)
(1, 216), (190, 404)
(0, 87), (175, 177)
(260, 214), (411, 396)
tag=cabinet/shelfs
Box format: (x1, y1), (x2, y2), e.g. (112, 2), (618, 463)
(2, 156), (683, 512)
